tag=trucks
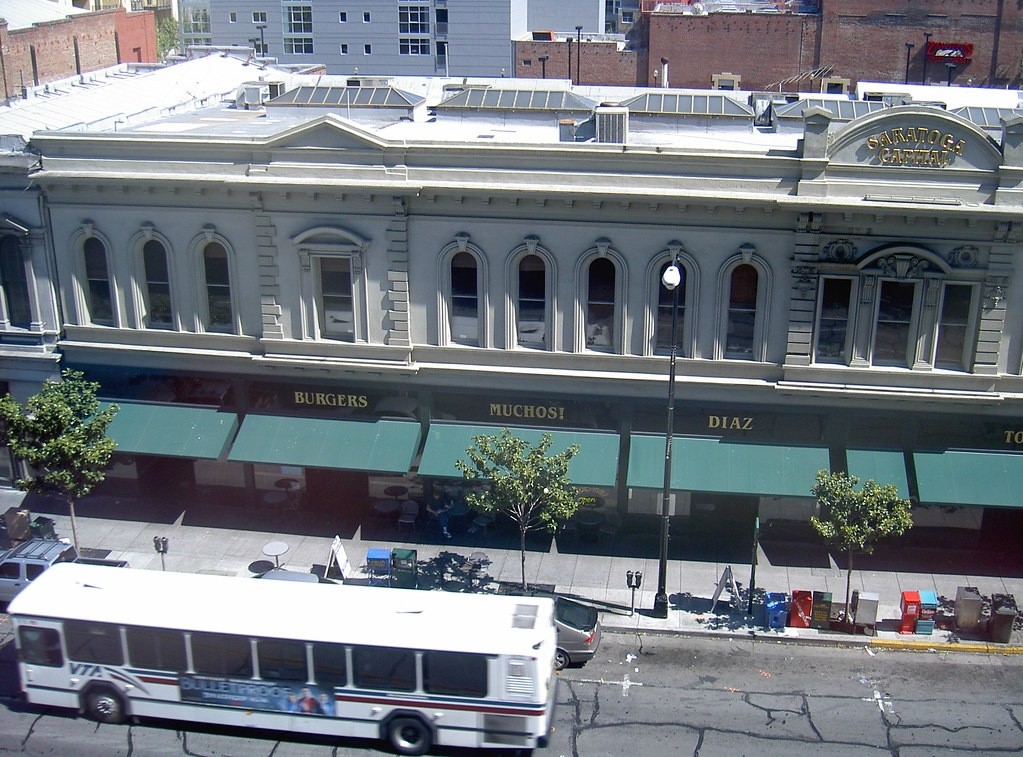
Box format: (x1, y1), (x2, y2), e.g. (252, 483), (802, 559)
(0, 538), (130, 602)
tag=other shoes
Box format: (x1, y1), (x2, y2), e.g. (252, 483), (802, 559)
(446, 533), (452, 539)
(443, 527), (447, 534)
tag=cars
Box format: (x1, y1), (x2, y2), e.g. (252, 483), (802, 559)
(495, 592), (601, 671)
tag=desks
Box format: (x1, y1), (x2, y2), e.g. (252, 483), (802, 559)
(275, 478), (298, 497)
(262, 541), (289, 572)
(263, 490), (289, 516)
(384, 487), (409, 501)
(573, 510), (602, 544)
(373, 500), (401, 526)
(447, 499), (469, 532)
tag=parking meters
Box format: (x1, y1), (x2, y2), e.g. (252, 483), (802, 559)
(626, 571), (642, 614)
(153, 536), (168, 571)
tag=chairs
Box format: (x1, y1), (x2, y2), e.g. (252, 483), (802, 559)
(397, 500), (418, 535)
(560, 513), (577, 539)
(283, 486), (310, 516)
(429, 511), (439, 524)
(598, 511), (623, 543)
(471, 511), (497, 539)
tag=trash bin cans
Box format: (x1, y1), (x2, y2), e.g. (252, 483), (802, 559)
(990, 593), (1018, 644)
(763, 589), (834, 629)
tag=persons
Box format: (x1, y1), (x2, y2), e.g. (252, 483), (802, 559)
(287, 686), (333, 714)
(426, 490), (455, 538)
(39, 634), (61, 662)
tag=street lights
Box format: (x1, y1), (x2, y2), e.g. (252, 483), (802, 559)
(575, 26), (583, 85)
(567, 37), (573, 79)
(249, 38), (262, 49)
(922, 33), (933, 85)
(500, 68), (505, 78)
(256, 25), (267, 57)
(905, 42), (914, 84)
(538, 56), (549, 79)
(653, 69), (659, 87)
(945, 63), (957, 87)
(652, 252), (689, 619)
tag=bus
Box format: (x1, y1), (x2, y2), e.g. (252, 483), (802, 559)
(6, 561), (557, 757)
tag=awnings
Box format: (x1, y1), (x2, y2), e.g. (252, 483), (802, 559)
(62, 396), (1023, 509)
